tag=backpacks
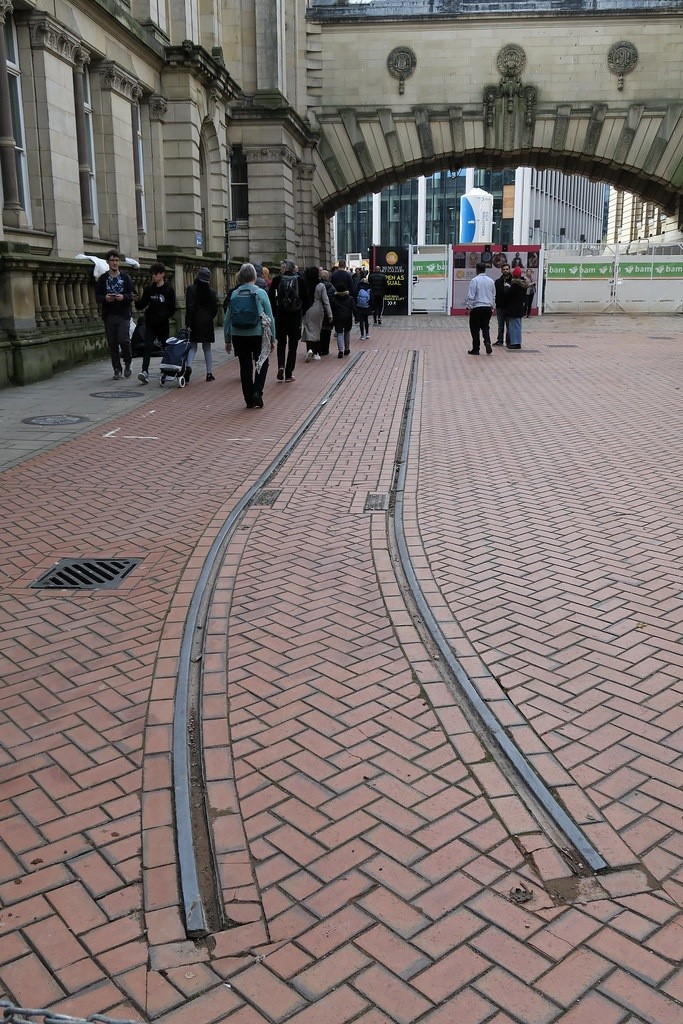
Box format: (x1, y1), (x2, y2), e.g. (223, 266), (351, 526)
(357, 289), (371, 308)
(231, 287), (264, 330)
(278, 275), (302, 312)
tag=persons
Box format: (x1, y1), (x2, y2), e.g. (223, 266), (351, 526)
(492, 264), (527, 349)
(223, 259), (388, 382)
(94, 251), (177, 384)
(184, 268), (219, 383)
(524, 269), (536, 318)
(224, 262), (276, 408)
(496, 252), (539, 268)
(466, 262), (496, 355)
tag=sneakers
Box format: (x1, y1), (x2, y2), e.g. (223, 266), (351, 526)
(113, 368), (122, 379)
(124, 362), (133, 377)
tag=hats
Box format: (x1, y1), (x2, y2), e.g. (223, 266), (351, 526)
(195, 267), (211, 283)
(512, 266), (522, 277)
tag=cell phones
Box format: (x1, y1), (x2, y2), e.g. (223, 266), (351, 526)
(108, 294), (116, 296)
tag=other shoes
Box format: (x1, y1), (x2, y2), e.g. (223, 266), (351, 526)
(285, 375), (296, 381)
(242, 380), (253, 408)
(336, 282), (347, 292)
(277, 368), (284, 380)
(492, 341), (503, 347)
(313, 352), (321, 360)
(251, 381), (263, 408)
(206, 373), (215, 381)
(344, 350), (350, 355)
(486, 344), (492, 353)
(365, 334), (370, 338)
(468, 349), (479, 355)
(138, 371), (150, 385)
(305, 349), (313, 362)
(184, 367), (192, 382)
(338, 352), (343, 358)
(360, 336), (364, 339)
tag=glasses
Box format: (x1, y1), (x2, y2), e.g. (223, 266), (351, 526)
(109, 259), (119, 262)
